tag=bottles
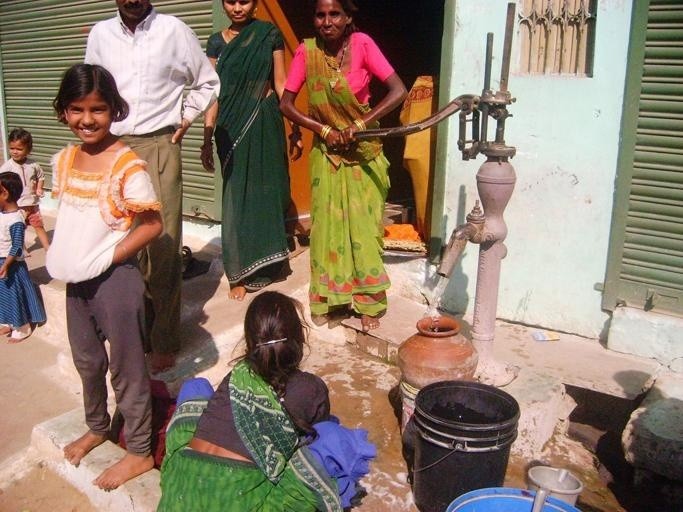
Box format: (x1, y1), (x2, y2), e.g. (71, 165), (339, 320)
(396, 313), (477, 391)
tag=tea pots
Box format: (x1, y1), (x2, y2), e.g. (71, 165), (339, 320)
(525, 464), (583, 508)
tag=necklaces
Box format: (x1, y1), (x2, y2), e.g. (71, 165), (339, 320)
(228, 25), (240, 36)
(322, 40), (346, 73)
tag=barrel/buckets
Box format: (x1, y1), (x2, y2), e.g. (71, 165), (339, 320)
(409, 380), (521, 511)
(445, 486), (583, 512)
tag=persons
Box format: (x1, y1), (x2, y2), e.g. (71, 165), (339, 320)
(0, 171), (46, 343)
(43, 63), (163, 491)
(278, 0), (407, 331)
(0, 127), (49, 258)
(156, 292), (375, 511)
(84, 0), (220, 372)
(201, 0), (304, 301)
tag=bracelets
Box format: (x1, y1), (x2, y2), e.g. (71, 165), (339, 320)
(353, 118), (366, 132)
(288, 131), (302, 139)
(121, 244), (130, 261)
(200, 142), (212, 150)
(319, 125), (333, 140)
